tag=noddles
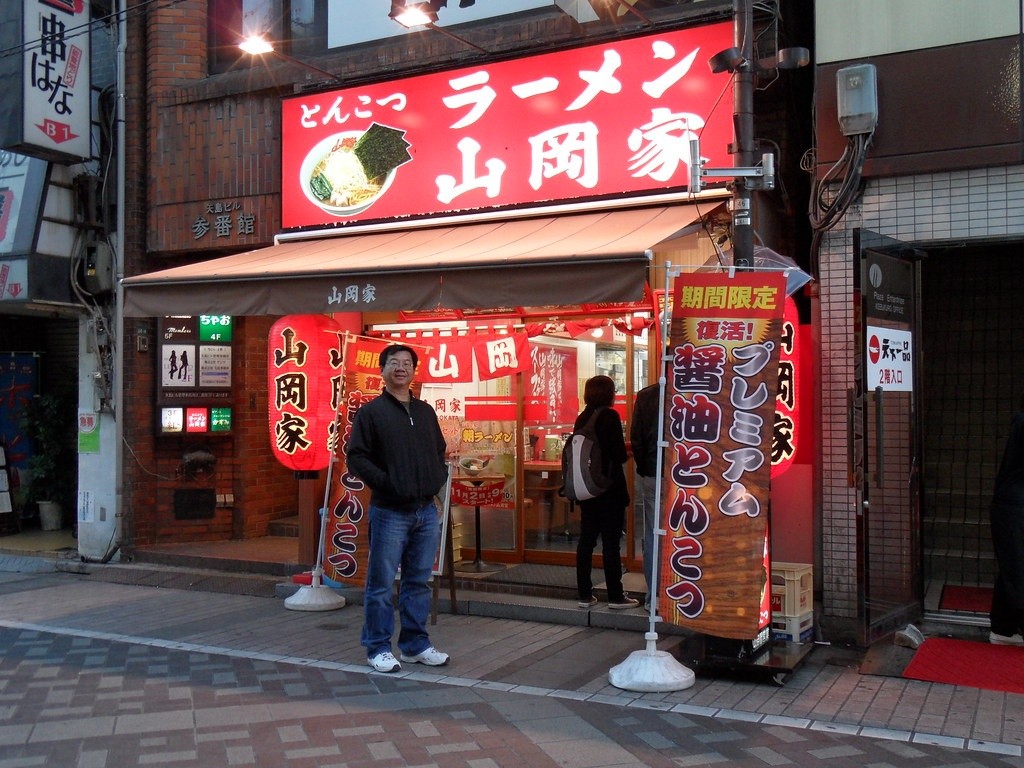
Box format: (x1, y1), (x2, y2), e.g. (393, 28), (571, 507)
(315, 148), (380, 206)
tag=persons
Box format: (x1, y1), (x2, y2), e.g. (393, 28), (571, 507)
(630, 382), (660, 614)
(572, 375), (640, 609)
(989, 392), (1024, 648)
(346, 344), (451, 672)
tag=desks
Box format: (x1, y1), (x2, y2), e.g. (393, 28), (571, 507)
(525, 460), (577, 537)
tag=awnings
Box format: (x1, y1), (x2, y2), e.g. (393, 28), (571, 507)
(119, 200), (726, 318)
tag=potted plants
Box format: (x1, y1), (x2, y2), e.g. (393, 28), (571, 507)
(20, 395), (64, 531)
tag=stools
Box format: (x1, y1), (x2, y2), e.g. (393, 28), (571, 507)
(527, 480), (567, 545)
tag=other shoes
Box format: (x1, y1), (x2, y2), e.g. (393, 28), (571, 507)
(644, 597), (659, 612)
(989, 631), (1024, 645)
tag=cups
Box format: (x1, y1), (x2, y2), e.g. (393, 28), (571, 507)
(544, 434), (559, 461)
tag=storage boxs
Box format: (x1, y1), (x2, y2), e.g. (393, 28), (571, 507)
(772, 562), (814, 642)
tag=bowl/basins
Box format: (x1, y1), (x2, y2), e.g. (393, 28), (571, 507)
(458, 458), (486, 475)
(298, 130), (398, 217)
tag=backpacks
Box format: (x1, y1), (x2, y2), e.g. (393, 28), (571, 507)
(558, 407), (612, 513)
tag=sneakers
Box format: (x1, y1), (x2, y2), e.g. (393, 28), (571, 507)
(400, 646), (450, 667)
(608, 592), (639, 609)
(367, 652), (401, 673)
(578, 595), (597, 608)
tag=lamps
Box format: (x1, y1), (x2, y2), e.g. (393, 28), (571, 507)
(389, 3), (493, 63)
(238, 32), (343, 85)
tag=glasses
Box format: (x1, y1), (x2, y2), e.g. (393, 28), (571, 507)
(386, 360), (414, 368)
(614, 389), (618, 393)
